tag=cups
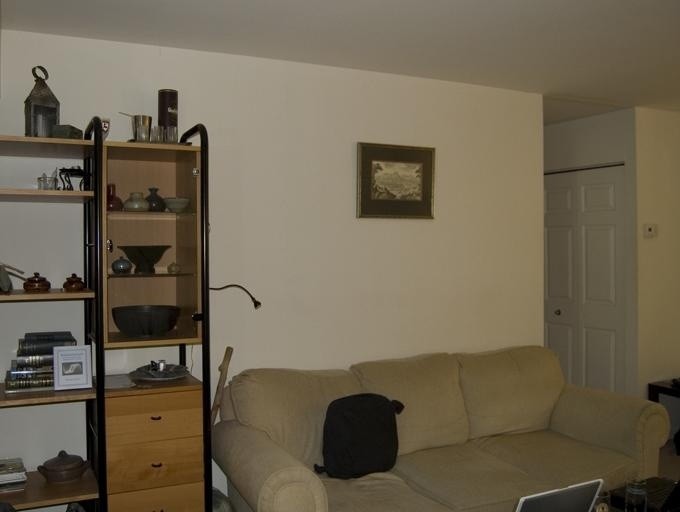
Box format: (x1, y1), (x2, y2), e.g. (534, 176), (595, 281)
(595, 491), (610, 511)
(36, 176), (58, 191)
(131, 89), (178, 143)
(622, 478), (648, 512)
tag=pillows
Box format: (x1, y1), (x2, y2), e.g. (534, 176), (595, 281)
(314, 393), (404, 480)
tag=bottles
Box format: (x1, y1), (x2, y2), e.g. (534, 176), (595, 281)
(23, 272), (84, 295)
(112, 256), (132, 276)
(107, 184), (123, 211)
(146, 187), (165, 213)
(167, 261), (182, 275)
(124, 192), (149, 213)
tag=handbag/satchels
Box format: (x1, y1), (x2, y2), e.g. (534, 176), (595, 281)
(324, 392), (398, 478)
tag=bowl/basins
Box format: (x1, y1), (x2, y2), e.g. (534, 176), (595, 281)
(163, 196), (189, 213)
(111, 305), (180, 338)
(118, 244), (173, 274)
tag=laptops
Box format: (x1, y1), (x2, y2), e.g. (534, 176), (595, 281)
(515, 479), (603, 512)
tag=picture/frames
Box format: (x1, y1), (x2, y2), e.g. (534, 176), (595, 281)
(353, 140), (435, 218)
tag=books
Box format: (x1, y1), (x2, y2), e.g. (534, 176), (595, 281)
(4, 331), (77, 394)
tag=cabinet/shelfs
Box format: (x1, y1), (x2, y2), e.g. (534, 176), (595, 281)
(0, 116), (216, 512)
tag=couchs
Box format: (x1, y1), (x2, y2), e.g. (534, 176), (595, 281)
(208, 342), (672, 512)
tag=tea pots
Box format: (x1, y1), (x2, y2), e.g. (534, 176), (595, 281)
(38, 450), (92, 485)
